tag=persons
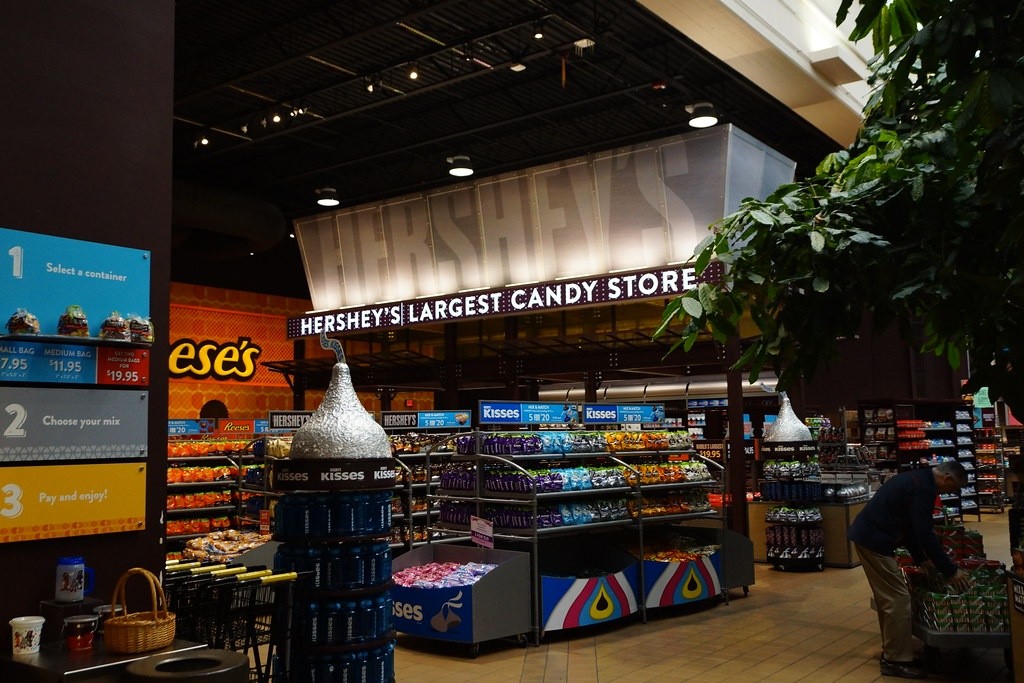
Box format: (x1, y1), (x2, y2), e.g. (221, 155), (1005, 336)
(846, 461), (972, 680)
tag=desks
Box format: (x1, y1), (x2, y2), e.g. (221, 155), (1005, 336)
(5, 629), (208, 683)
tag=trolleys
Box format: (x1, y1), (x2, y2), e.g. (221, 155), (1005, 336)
(165, 556), (298, 682)
(870, 506), (1014, 674)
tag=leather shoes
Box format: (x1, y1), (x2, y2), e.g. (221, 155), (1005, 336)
(879, 658), (928, 678)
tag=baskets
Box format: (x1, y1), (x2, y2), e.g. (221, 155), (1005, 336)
(103, 567), (176, 654)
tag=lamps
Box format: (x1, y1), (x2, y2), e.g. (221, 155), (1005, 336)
(447, 155), (474, 177)
(315, 188), (339, 207)
(510, 56), (526, 72)
(299, 104), (312, 114)
(685, 101), (718, 128)
(533, 19), (544, 40)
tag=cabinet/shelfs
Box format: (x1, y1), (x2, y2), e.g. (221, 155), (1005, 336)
(167, 400), (1007, 661)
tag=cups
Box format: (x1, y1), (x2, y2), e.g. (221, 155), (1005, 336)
(94, 604), (123, 634)
(9, 616), (45, 655)
(55, 555), (95, 602)
(64, 615), (99, 651)
